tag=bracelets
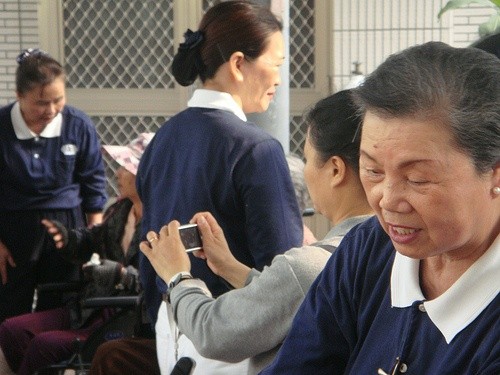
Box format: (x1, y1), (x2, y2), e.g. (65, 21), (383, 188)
(166, 272), (194, 304)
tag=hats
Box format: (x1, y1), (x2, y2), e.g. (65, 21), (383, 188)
(101, 132), (157, 177)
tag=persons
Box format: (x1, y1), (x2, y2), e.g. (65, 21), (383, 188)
(0, 133), (160, 375)
(136, 1), (319, 375)
(0, 49), (106, 316)
(255, 41), (500, 375)
(139, 88), (376, 375)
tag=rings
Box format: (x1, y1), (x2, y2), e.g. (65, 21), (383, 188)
(149, 237), (159, 243)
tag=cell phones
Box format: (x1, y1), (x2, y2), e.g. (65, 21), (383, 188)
(178, 223), (202, 252)
